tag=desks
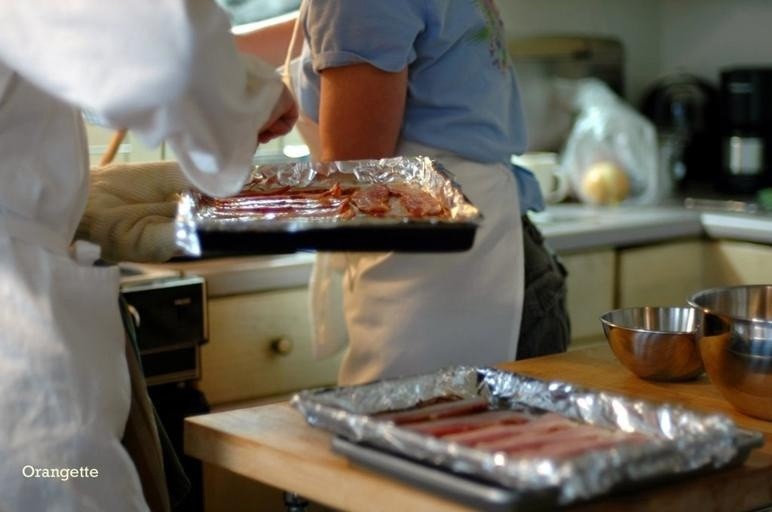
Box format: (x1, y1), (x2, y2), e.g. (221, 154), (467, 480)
(182, 335), (772, 510)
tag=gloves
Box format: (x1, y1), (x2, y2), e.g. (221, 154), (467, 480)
(75, 161), (192, 264)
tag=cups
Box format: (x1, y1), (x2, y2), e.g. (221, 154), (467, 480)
(512, 153), (569, 206)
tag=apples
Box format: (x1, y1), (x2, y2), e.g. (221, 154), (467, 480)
(582, 163), (630, 202)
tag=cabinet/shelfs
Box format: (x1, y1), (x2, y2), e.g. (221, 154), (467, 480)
(196, 288), (332, 510)
(559, 238), (770, 351)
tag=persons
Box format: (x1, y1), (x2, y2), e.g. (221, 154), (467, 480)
(230, 0), (571, 389)
(0, 0), (298, 512)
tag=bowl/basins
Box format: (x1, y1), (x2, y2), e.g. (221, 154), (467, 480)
(599, 305), (707, 383)
(685, 283), (772, 422)
